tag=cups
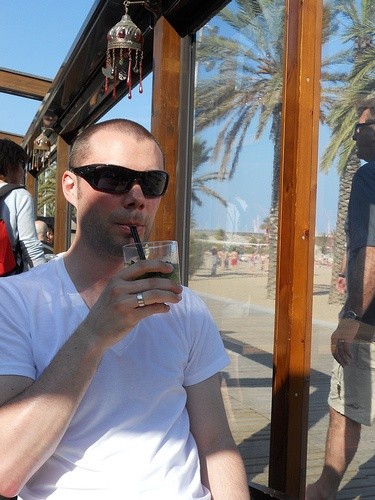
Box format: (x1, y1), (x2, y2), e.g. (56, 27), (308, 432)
(124, 240), (184, 300)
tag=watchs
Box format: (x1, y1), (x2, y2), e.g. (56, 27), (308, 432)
(340, 311), (362, 322)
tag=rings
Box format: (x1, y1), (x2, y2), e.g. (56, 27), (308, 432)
(137, 293), (145, 307)
(338, 339), (345, 343)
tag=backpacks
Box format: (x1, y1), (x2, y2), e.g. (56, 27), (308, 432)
(0, 184), (27, 276)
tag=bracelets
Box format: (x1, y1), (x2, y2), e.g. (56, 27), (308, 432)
(339, 274), (346, 278)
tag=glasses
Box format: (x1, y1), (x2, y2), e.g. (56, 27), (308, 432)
(69, 164), (169, 198)
(354, 121), (375, 132)
(19, 161), (25, 169)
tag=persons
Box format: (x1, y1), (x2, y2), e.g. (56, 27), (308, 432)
(306, 97), (375, 500)
(0, 119), (251, 500)
(209, 244), (257, 276)
(0, 139), (54, 277)
